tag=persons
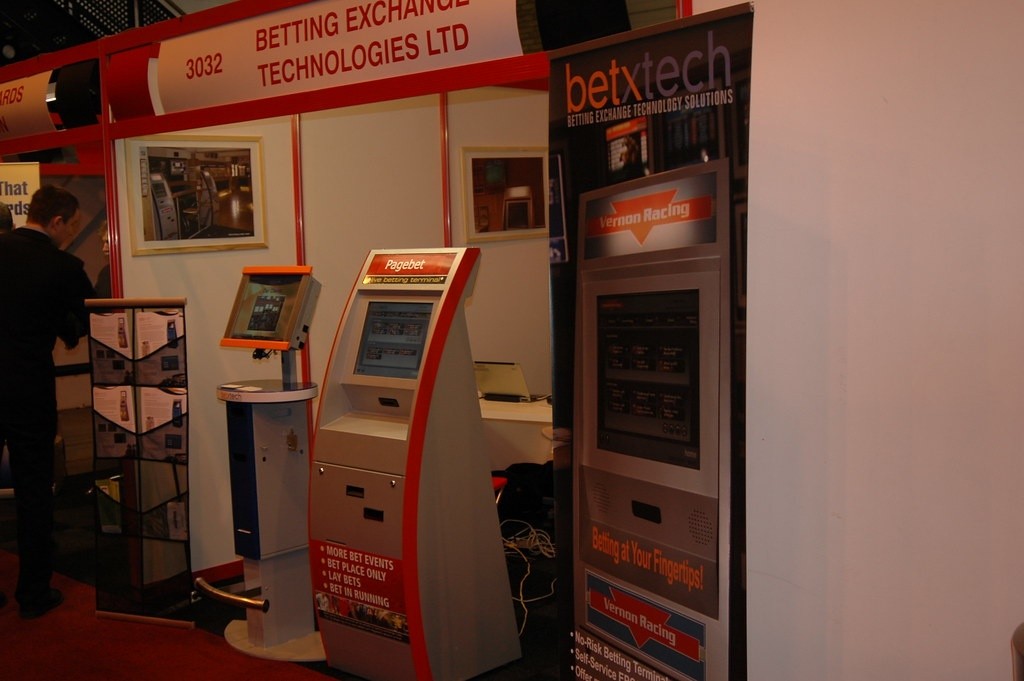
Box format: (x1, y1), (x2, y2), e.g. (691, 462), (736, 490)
(0, 186), (115, 617)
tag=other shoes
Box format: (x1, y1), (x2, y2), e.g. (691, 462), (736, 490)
(0, 592), (7, 608)
(20, 589), (61, 618)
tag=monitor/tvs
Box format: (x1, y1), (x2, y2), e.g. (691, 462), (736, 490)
(353, 302), (433, 380)
(595, 289), (700, 471)
(153, 183), (167, 198)
(220, 268), (322, 350)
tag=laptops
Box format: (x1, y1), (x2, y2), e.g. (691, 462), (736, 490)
(474, 361), (552, 403)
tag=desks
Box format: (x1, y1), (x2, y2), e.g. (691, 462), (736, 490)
(478, 395), (552, 475)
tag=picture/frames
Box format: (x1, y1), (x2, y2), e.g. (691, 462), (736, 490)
(461, 147), (548, 244)
(124, 134), (268, 257)
